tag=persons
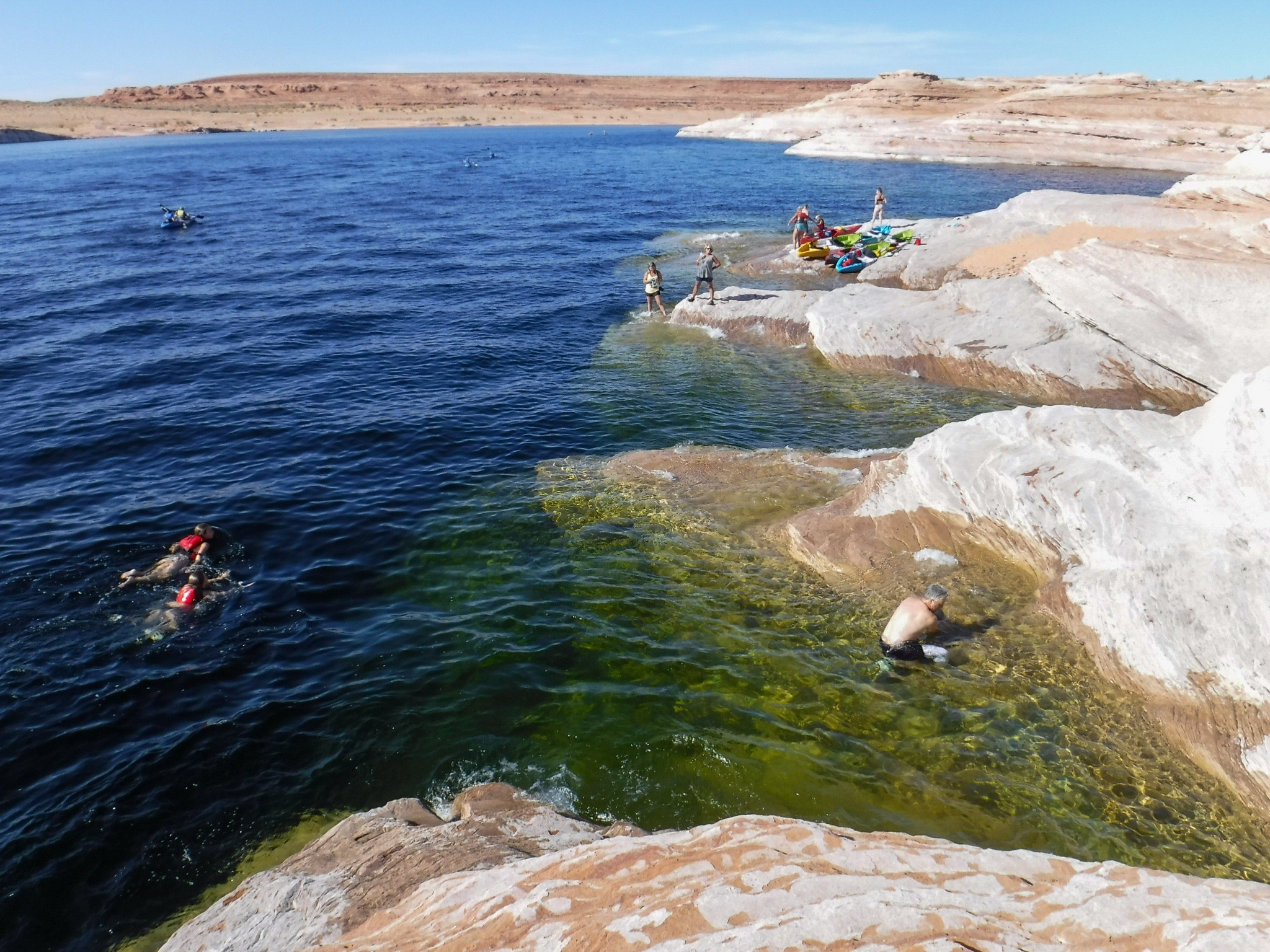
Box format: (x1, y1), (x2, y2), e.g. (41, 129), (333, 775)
(788, 203), (824, 250)
(686, 243), (721, 305)
(869, 187), (887, 229)
(879, 584), (948, 660)
(118, 522), (231, 624)
(166, 207), (189, 223)
(643, 262), (667, 317)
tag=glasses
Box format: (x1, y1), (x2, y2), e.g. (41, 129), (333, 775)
(707, 248), (712, 250)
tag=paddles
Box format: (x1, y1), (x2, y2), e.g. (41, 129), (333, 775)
(162, 207), (205, 219)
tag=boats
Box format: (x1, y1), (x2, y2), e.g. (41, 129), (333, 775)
(836, 240), (890, 273)
(800, 223), (862, 245)
(797, 232), (861, 258)
(825, 247), (858, 265)
(886, 228), (913, 253)
(859, 224), (891, 243)
(161, 215), (196, 229)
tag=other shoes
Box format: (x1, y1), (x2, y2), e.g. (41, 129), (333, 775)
(686, 297), (695, 302)
(707, 300), (714, 305)
